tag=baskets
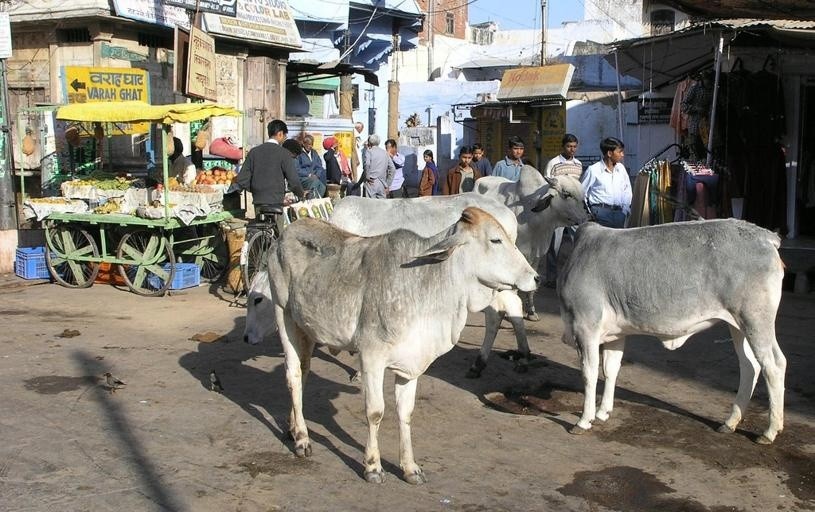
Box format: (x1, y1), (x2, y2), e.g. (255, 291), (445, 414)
(14, 245), (201, 291)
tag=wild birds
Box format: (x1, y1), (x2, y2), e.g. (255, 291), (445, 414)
(208, 368), (225, 391)
(103, 372), (127, 394)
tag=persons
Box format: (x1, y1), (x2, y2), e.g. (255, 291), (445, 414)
(162, 134), (199, 250)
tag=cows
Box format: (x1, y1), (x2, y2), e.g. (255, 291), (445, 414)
(555, 216), (788, 445)
(264, 206), (542, 486)
(470, 164), (591, 323)
(242, 190), (530, 382)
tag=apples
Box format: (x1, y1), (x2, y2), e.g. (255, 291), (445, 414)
(195, 167), (235, 185)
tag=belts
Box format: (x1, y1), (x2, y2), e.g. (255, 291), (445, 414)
(591, 203), (623, 211)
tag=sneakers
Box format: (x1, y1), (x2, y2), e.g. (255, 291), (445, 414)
(544, 280), (556, 289)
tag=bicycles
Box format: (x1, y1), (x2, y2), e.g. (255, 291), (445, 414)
(238, 202), (283, 293)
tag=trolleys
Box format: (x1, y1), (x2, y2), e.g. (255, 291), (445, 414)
(22, 101), (248, 297)
